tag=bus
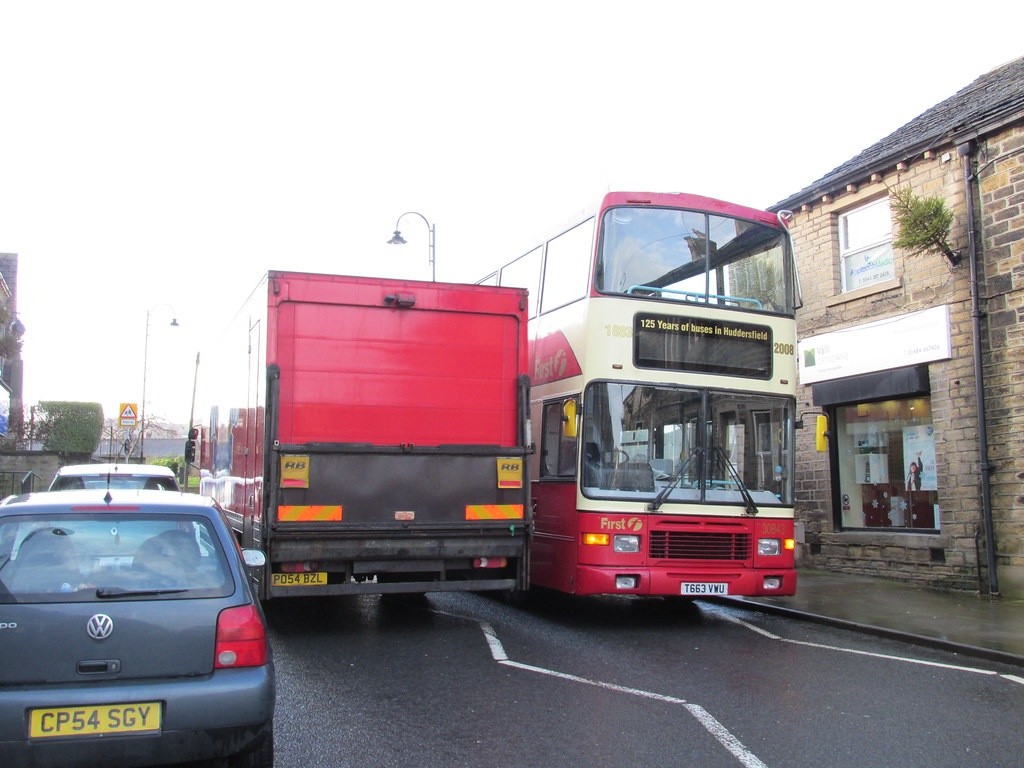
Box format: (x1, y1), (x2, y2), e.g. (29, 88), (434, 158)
(468, 190), (833, 607)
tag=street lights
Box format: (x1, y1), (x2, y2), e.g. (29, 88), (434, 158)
(386, 212), (438, 284)
(140, 298), (181, 461)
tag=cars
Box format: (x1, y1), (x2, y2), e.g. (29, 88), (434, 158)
(7, 460), (198, 574)
(0, 490), (275, 768)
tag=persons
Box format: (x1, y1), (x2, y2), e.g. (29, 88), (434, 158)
(905, 450), (923, 491)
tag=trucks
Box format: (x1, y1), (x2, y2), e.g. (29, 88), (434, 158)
(185, 265), (534, 612)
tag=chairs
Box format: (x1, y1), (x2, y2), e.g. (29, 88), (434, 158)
(57, 477), (86, 490)
(144, 477), (175, 491)
(11, 526), (202, 597)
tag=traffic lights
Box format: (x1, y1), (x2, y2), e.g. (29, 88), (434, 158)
(184, 440), (196, 463)
(189, 429), (199, 441)
